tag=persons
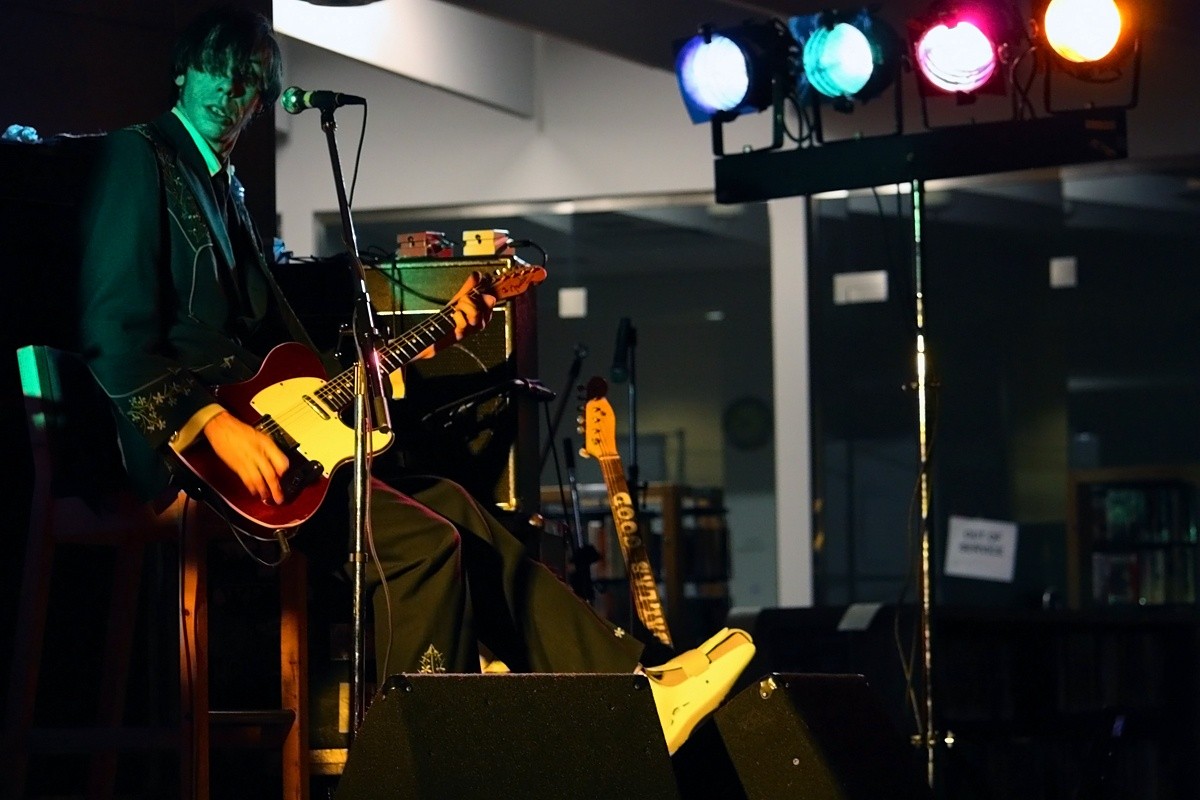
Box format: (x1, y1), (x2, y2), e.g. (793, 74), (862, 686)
(78, 14), (756, 756)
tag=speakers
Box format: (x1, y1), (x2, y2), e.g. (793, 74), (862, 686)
(360, 255), (540, 515)
(712, 673), (921, 800)
(337, 673), (680, 800)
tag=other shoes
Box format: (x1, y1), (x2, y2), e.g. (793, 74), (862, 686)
(645, 627), (756, 757)
(315, 749), (349, 776)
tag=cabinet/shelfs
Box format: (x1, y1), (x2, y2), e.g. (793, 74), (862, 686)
(1065, 462), (1200, 610)
(535, 485), (728, 649)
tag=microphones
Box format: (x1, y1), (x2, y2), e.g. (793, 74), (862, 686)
(609, 318), (633, 383)
(280, 87), (366, 114)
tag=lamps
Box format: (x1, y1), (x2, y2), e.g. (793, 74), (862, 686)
(678, 1), (1149, 126)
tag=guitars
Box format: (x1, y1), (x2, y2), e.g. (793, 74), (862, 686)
(574, 374), (675, 655)
(164, 255), (548, 542)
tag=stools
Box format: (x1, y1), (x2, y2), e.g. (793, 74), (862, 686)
(14, 346), (312, 800)
